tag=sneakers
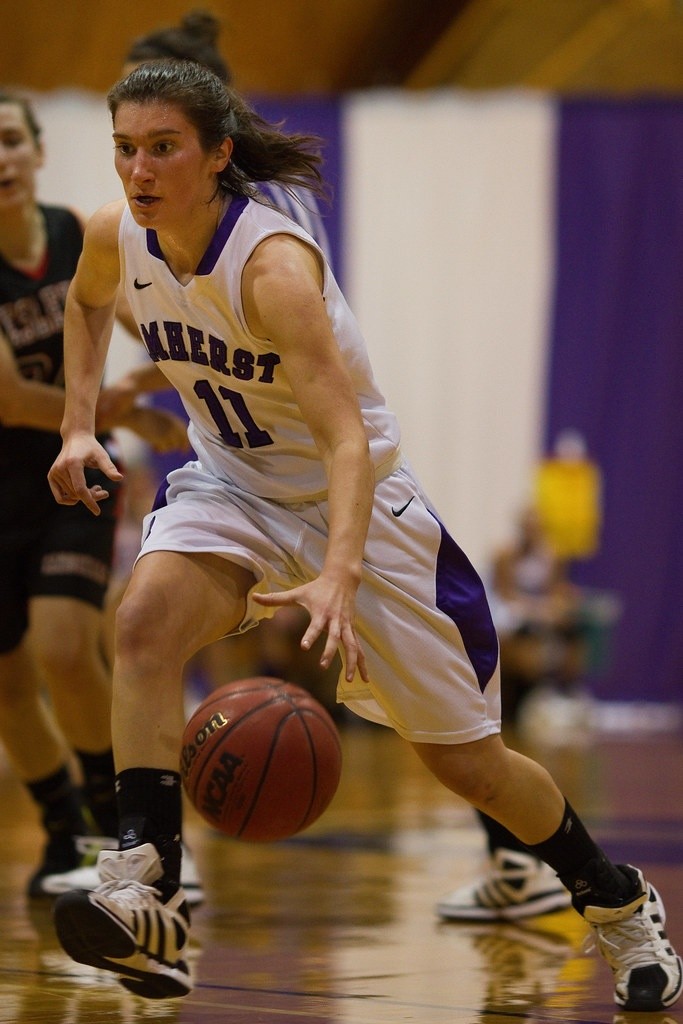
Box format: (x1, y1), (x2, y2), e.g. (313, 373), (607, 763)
(437, 849), (572, 916)
(42, 848), (203, 902)
(440, 922), (572, 1005)
(573, 864), (683, 1011)
(53, 844), (194, 999)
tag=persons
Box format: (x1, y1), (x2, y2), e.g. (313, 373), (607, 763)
(492, 431), (613, 687)
(0, 13), (573, 917)
(51, 61), (683, 1010)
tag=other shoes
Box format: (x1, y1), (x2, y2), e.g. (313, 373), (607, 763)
(27, 844), (88, 900)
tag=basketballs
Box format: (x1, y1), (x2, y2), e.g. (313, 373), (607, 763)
(179, 675), (345, 845)
